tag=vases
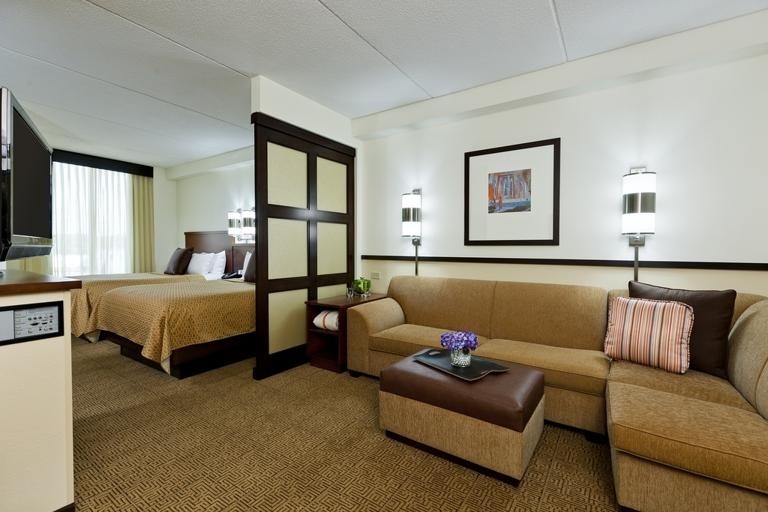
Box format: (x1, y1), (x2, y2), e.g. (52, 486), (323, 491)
(450, 347), (470, 370)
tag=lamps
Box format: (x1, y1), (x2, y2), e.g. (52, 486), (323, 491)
(226, 209), (243, 236)
(400, 188), (424, 276)
(240, 211), (256, 239)
(620, 166), (659, 285)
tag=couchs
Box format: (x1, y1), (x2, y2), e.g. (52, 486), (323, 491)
(343, 272), (768, 510)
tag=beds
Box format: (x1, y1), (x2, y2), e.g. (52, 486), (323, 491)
(98, 244), (261, 379)
(71, 231), (236, 341)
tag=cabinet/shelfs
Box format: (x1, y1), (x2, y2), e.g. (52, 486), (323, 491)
(304, 290), (390, 374)
(0, 268), (84, 511)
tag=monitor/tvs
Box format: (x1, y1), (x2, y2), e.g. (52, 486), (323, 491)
(0, 87), (52, 276)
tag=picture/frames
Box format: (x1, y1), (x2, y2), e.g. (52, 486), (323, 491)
(462, 136), (561, 248)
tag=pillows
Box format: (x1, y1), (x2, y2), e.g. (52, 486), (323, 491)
(186, 251), (215, 277)
(601, 294), (693, 377)
(244, 249), (257, 283)
(627, 280), (738, 382)
(211, 249), (227, 278)
(163, 246), (194, 275)
(242, 251), (251, 279)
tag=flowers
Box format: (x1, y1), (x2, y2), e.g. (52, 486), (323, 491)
(440, 327), (481, 353)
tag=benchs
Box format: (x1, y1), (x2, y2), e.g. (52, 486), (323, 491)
(376, 347), (548, 485)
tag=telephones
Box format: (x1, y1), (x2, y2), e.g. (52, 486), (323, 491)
(222, 272), (242, 279)
